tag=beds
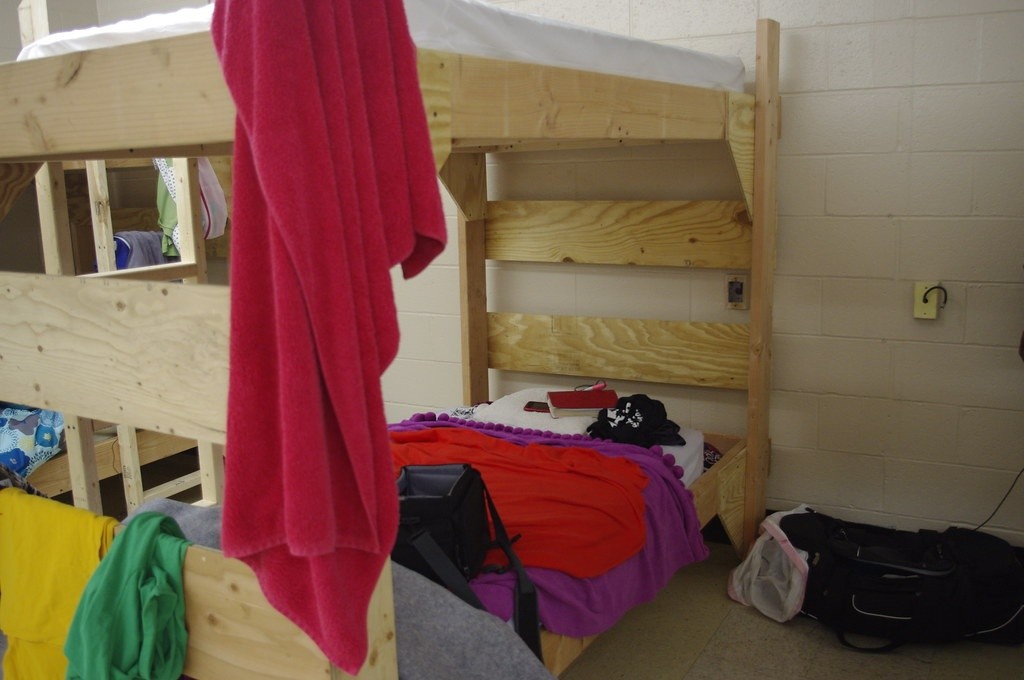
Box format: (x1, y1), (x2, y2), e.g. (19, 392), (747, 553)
(0, 0), (746, 175)
(119, 401), (747, 680)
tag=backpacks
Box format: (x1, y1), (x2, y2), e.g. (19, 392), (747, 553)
(780, 511), (1023, 653)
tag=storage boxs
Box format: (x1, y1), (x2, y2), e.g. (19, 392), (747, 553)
(393, 460), (487, 590)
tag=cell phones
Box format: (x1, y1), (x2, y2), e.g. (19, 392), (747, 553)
(525, 401), (550, 414)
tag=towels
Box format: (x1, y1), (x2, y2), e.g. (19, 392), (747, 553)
(206, 0), (451, 679)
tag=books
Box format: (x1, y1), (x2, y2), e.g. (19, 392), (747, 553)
(546, 390), (619, 419)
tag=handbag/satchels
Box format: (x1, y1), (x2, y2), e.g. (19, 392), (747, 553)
(393, 463), (546, 665)
